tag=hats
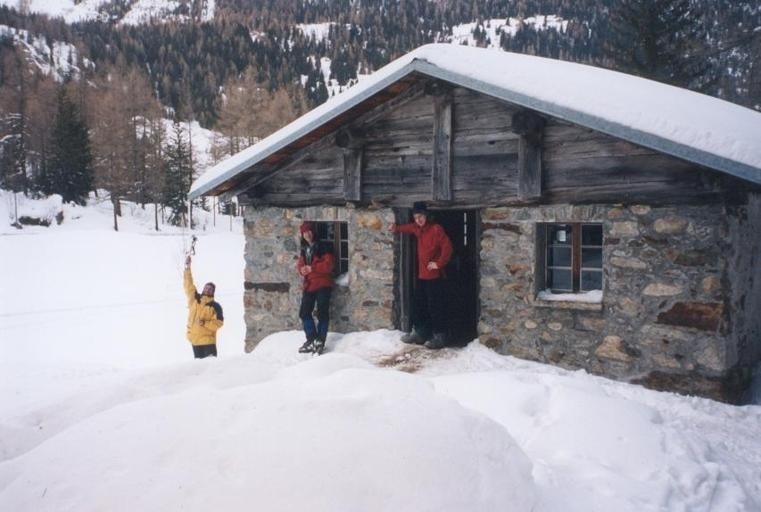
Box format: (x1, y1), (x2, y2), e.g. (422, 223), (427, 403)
(411, 200), (430, 216)
(300, 221), (313, 233)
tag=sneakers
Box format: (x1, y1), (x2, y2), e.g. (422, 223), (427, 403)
(297, 337), (325, 355)
(424, 332), (449, 349)
(401, 325), (426, 344)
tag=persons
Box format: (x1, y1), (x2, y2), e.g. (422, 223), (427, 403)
(183, 253), (225, 360)
(388, 201), (454, 349)
(297, 221), (336, 355)
